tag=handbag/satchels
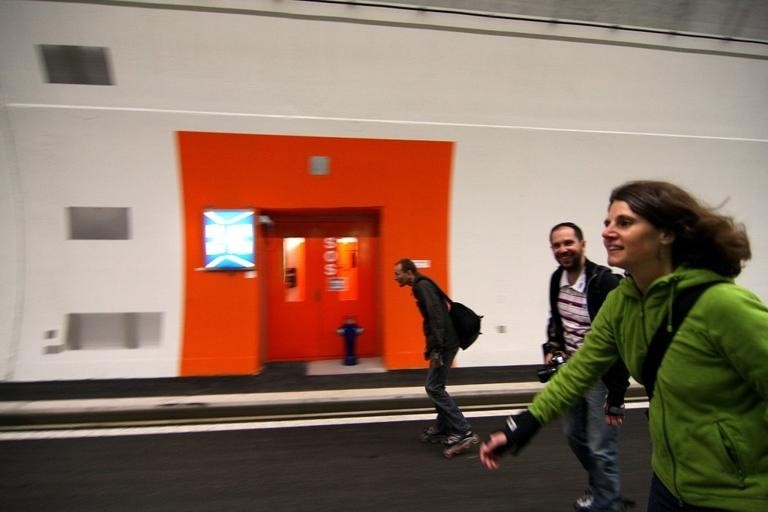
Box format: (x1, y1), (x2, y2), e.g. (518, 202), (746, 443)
(448, 303), (481, 349)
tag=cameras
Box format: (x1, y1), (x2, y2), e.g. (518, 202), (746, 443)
(537, 351), (566, 383)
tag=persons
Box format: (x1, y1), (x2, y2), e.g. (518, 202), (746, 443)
(394, 258), (473, 447)
(480, 181), (768, 512)
(541, 222), (630, 512)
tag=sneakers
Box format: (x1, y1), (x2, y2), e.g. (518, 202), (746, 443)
(577, 493), (594, 508)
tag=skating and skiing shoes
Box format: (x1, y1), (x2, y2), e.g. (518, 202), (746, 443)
(422, 427), (479, 458)
